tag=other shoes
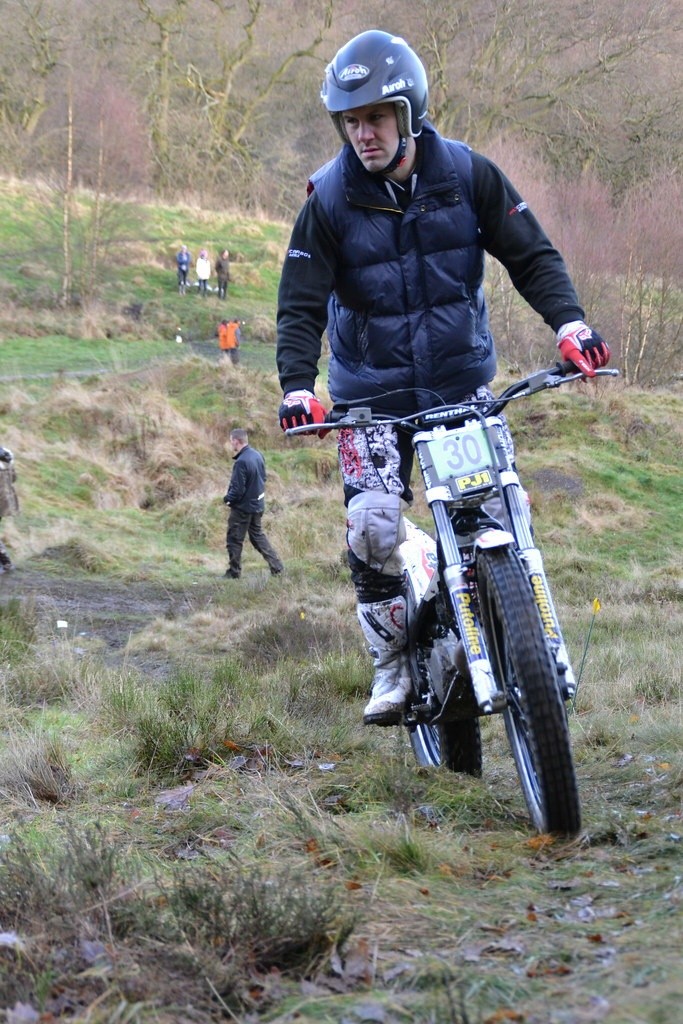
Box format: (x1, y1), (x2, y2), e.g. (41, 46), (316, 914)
(215, 574), (232, 581)
(0, 563), (14, 575)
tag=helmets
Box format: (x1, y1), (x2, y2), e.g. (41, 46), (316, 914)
(320, 30), (428, 136)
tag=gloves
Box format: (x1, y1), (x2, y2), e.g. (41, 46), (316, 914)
(556, 320), (610, 383)
(278, 389), (332, 439)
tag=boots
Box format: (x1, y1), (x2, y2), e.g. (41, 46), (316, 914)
(355, 568), (416, 724)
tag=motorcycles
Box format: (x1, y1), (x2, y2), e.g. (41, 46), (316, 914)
(281, 354), (620, 842)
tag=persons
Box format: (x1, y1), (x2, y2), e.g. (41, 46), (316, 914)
(276, 31), (611, 726)
(196, 250), (211, 299)
(216, 319), (243, 364)
(215, 250), (232, 299)
(176, 245), (192, 296)
(222, 429), (283, 579)
(0, 447), (19, 575)
